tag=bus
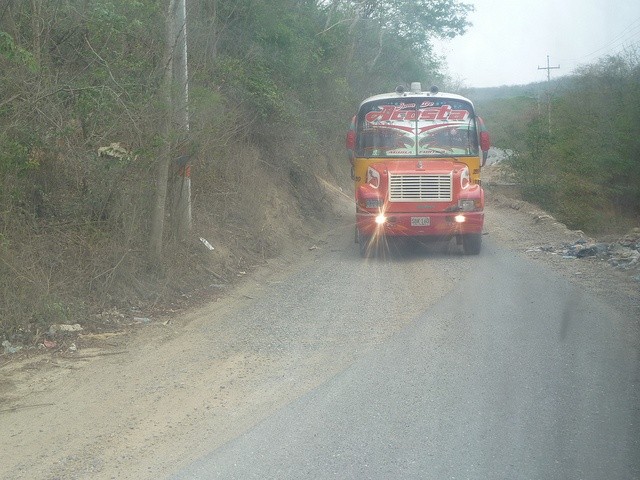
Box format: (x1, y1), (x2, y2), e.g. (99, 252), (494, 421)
(347, 83), (491, 259)
